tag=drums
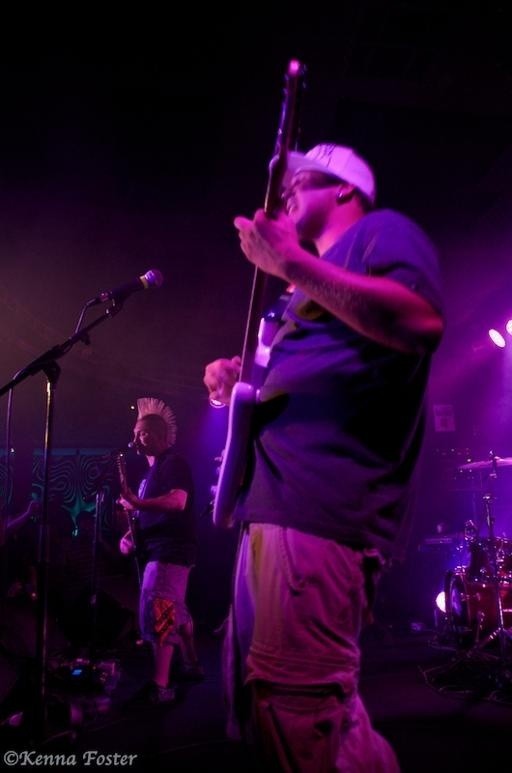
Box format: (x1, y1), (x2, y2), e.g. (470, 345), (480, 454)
(445, 570), (512, 639)
(468, 536), (512, 582)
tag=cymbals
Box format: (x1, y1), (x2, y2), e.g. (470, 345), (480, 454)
(459, 457), (512, 469)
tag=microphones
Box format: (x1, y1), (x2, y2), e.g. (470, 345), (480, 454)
(92, 267), (170, 305)
(107, 441), (137, 462)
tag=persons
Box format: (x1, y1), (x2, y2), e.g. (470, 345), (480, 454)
(116, 412), (205, 689)
(203, 137), (444, 771)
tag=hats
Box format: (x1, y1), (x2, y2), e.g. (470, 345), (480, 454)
(268, 142), (376, 206)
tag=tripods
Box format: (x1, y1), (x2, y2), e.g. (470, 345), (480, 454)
(465, 528), (512, 662)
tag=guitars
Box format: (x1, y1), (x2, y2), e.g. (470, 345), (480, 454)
(211, 76), (296, 528)
(116, 453), (143, 586)
